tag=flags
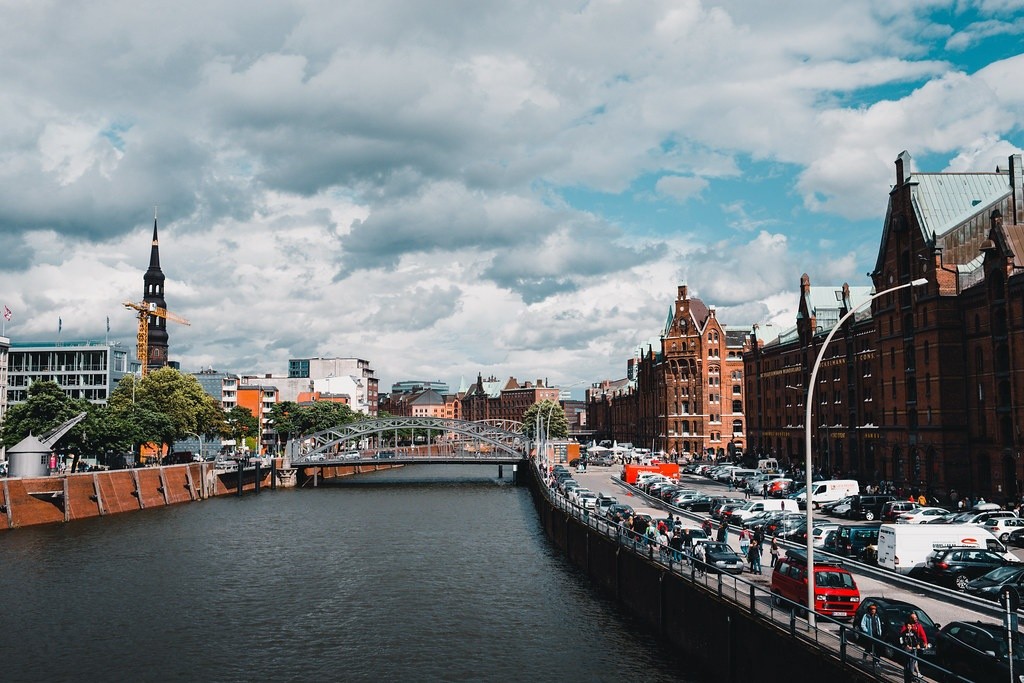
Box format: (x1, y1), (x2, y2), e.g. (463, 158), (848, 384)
(4, 307), (11, 321)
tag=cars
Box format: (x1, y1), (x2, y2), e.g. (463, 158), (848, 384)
(543, 445), (843, 549)
(926, 513), (963, 524)
(370, 450), (395, 459)
(694, 541), (744, 575)
(305, 453), (325, 462)
(821, 496), (851, 519)
(963, 563), (1024, 609)
(895, 506), (950, 524)
(851, 596), (942, 660)
(1008, 528), (1024, 546)
(339, 451), (361, 462)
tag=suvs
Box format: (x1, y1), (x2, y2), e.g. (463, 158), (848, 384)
(919, 620), (1024, 683)
(949, 503), (1018, 527)
(850, 494), (898, 521)
(878, 500), (924, 523)
(926, 546), (1011, 592)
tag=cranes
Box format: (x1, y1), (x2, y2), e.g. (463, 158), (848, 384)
(121, 296), (193, 378)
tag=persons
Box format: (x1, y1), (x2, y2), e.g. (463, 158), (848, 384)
(975, 493), (1024, 519)
(619, 455), (640, 465)
(59, 460), (66, 474)
(596, 453), (598, 458)
(539, 463), (582, 507)
(612, 509), (781, 579)
(666, 451), (970, 524)
(227, 449), (247, 457)
(582, 458), (588, 473)
(78, 461), (105, 472)
(859, 601), (882, 670)
(900, 612), (928, 683)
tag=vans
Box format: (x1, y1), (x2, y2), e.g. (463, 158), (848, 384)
(795, 480), (859, 511)
(875, 524), (1020, 575)
(771, 549), (860, 621)
(984, 517), (1024, 544)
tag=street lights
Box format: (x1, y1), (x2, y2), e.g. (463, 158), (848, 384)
(537, 394), (553, 465)
(185, 431), (203, 464)
(804, 276), (928, 635)
(546, 379), (587, 481)
